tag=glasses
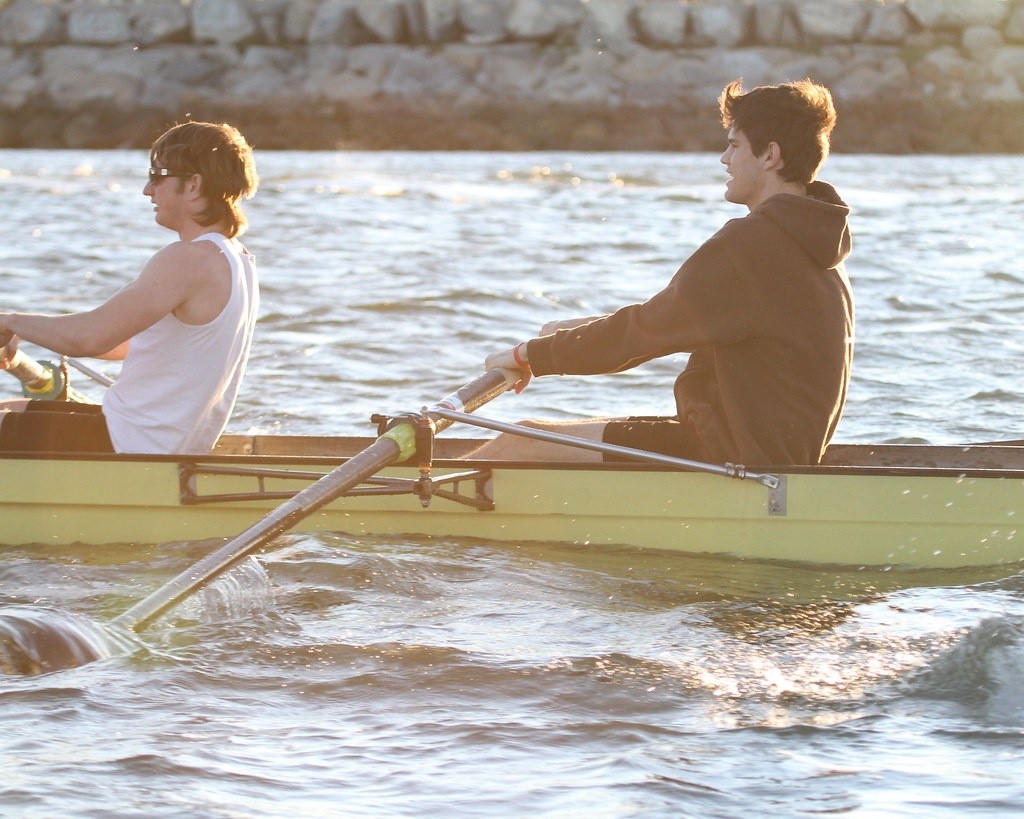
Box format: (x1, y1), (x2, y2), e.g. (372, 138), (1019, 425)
(149, 166), (197, 186)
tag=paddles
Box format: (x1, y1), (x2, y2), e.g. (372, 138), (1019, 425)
(0, 366), (525, 674)
(1, 345), (118, 405)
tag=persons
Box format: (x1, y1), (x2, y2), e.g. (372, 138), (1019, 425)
(455, 78), (855, 465)
(0, 121), (259, 454)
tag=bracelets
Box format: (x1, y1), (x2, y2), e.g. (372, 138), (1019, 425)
(514, 342), (529, 367)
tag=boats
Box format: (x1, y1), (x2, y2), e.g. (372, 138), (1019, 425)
(0, 435), (1024, 570)
